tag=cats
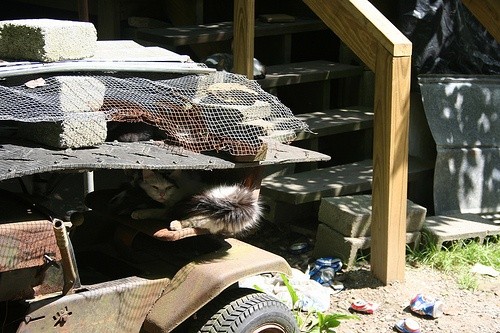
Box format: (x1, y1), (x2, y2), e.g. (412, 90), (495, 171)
(131, 169), (263, 234)
